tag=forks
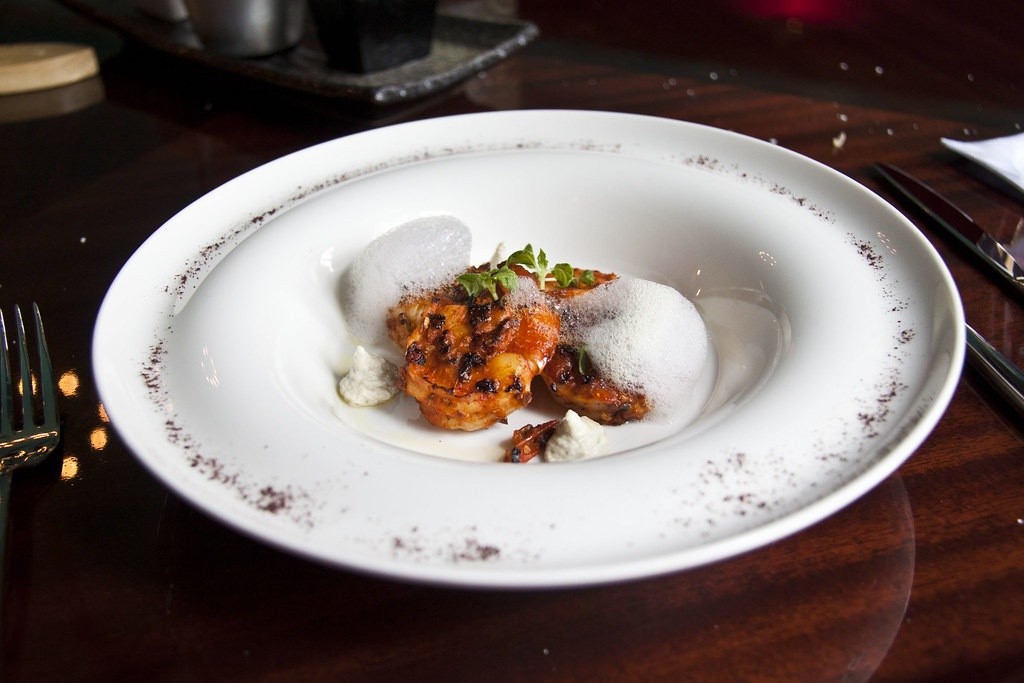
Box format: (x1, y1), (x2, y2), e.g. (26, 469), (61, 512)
(0, 303), (61, 547)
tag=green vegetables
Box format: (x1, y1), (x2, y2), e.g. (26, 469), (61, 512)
(453, 242), (596, 299)
(579, 349), (594, 376)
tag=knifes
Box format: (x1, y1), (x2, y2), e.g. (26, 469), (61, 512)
(864, 146), (1023, 304)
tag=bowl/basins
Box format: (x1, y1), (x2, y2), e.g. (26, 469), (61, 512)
(179, 0), (303, 59)
(308, 0), (436, 73)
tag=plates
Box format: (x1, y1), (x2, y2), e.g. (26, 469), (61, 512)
(67, 5), (543, 106)
(91, 108), (965, 585)
